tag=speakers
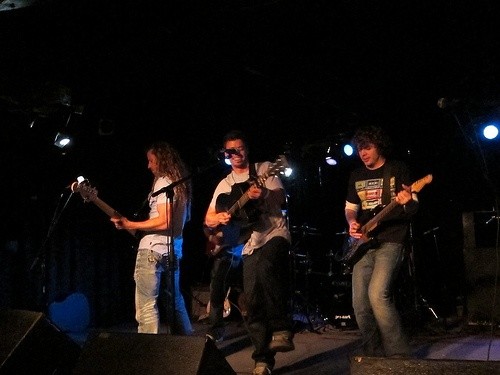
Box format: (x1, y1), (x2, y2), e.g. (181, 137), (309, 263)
(346, 354), (500, 375)
(0, 307), (83, 375)
(192, 287), (212, 320)
(72, 331), (237, 375)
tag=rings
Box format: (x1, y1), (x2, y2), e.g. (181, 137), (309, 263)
(117, 220), (122, 224)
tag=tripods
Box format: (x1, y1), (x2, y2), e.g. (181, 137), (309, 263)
(290, 251), (314, 331)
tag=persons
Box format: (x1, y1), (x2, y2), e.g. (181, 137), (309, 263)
(345, 127), (419, 359)
(111, 141), (194, 336)
(198, 133), (296, 375)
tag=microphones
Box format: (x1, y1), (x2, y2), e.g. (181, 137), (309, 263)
(197, 301), (203, 306)
(224, 148), (240, 155)
(65, 179), (90, 190)
(438, 98), (460, 108)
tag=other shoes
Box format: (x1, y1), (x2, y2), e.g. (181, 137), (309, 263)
(253, 362), (272, 375)
(206, 334), (223, 344)
(268, 330), (295, 353)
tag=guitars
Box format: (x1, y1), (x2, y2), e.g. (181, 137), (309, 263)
(214, 155), (291, 233)
(209, 225), (243, 255)
(72, 179), (150, 238)
(336, 173), (433, 268)
